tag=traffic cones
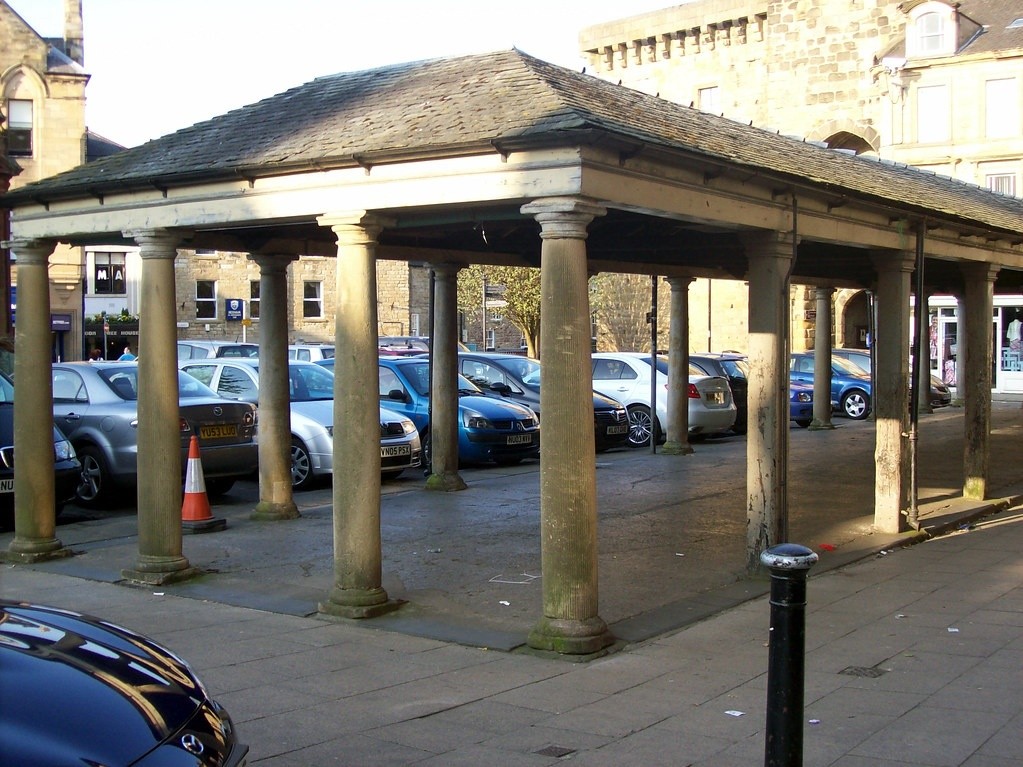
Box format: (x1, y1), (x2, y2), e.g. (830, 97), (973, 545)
(179, 434), (227, 535)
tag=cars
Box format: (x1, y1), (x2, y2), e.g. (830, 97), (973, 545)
(723, 352), (833, 427)
(5, 359), (259, 510)
(298, 355), (540, 475)
(178, 356), (423, 492)
(286, 333), (478, 389)
(660, 348), (747, 437)
(0, 366), (84, 535)
(0, 600), (249, 767)
(804, 348), (951, 412)
(509, 350), (737, 449)
(411, 355), (630, 459)
(788, 352), (872, 421)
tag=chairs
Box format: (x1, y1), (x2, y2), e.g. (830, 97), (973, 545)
(487, 362), (523, 388)
(403, 367), (418, 389)
(112, 377), (136, 400)
(53, 379), (76, 400)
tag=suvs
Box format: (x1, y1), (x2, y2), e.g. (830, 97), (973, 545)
(109, 338), (260, 400)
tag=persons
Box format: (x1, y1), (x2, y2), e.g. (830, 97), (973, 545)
(117, 346), (136, 361)
(929, 315), (938, 356)
(89, 349), (104, 362)
(1006, 318), (1023, 358)
